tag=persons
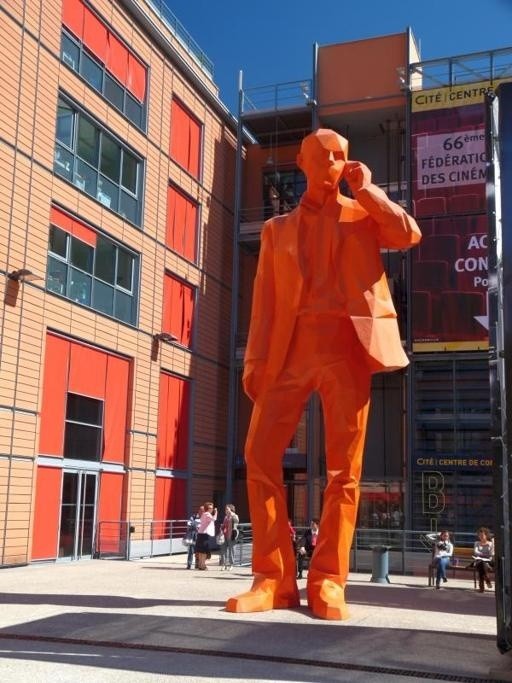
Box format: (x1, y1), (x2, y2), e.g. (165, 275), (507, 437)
(182, 504), (205, 569)
(368, 501), (403, 528)
(471, 524), (496, 593)
(426, 529), (455, 589)
(296, 516), (320, 580)
(224, 127), (422, 617)
(288, 519), (296, 558)
(195, 500), (218, 571)
(215, 503), (240, 571)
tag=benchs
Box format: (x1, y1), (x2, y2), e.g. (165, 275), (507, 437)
(426, 547), (498, 590)
(295, 536), (311, 561)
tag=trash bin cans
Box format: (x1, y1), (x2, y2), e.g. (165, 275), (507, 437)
(368, 545), (392, 584)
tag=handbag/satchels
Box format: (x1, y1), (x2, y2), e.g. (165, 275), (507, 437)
(233, 529), (239, 539)
(183, 538), (195, 545)
(216, 533), (225, 545)
(195, 553), (211, 559)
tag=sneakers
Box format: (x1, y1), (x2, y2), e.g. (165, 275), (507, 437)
(435, 577), (447, 589)
(480, 580), (492, 593)
(297, 572), (302, 579)
(187, 562), (233, 571)
